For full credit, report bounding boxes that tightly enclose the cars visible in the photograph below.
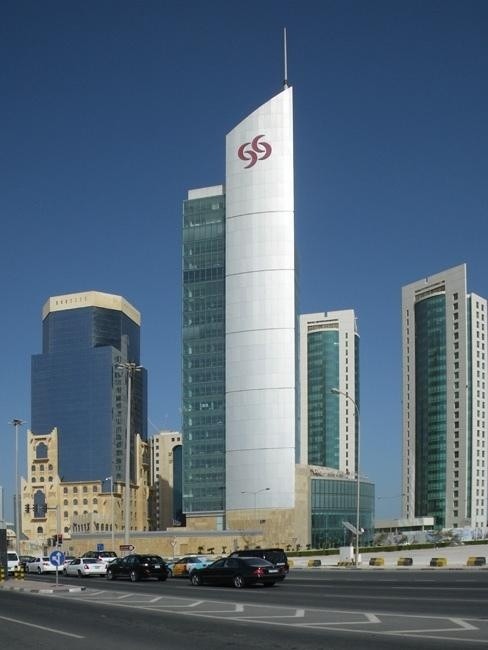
[188,557,285,588]
[7,551,231,582]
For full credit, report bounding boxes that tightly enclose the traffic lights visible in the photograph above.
[47,538,51,547]
[25,504,29,512]
[52,535,57,543]
[33,504,38,513]
[53,538,56,546]
[42,503,47,512]
[58,535,63,544]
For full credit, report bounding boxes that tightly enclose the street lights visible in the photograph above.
[105,475,115,552]
[330,389,361,569]
[240,487,271,548]
[377,493,406,545]
[8,418,27,556]
[116,362,144,557]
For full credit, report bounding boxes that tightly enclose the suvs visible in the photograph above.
[228,548,289,583]
